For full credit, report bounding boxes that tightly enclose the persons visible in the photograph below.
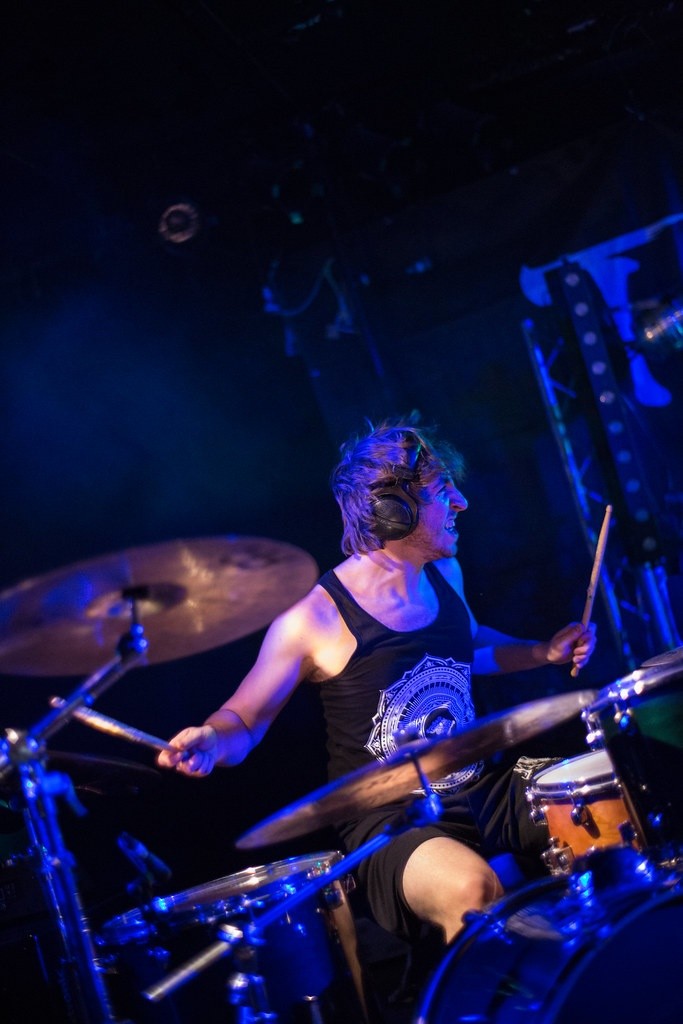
[152,419,599,945]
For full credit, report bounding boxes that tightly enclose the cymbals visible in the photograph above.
[1,539,319,677]
[234,692,594,850]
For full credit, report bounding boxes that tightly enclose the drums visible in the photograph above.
[412,849,683,1024]
[74,851,365,1024]
[525,748,647,876]
[587,646,683,868]
